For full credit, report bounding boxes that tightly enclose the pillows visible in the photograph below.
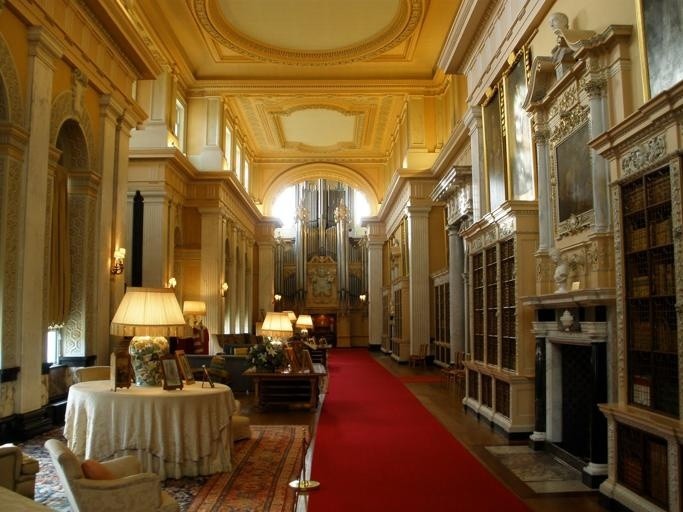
[81,460,116,480]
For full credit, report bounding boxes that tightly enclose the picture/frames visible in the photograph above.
[159,353,184,391]
[481,45,594,241]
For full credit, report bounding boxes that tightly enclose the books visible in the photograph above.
[623,175,677,416]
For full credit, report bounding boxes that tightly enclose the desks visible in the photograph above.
[62,380,237,481]
[241,363,327,412]
[309,344,333,367]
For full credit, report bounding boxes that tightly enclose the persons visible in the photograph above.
[549,247,568,293]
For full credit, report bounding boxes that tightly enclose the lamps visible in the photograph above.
[110,246,314,387]
[359,291,369,305]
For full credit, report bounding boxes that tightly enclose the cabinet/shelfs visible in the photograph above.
[460,199,539,441]
[380,276,410,362]
[587,80,683,512]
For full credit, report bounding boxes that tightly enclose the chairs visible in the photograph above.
[0,443,40,500]
[409,343,428,369]
[440,352,467,398]
[231,399,252,443]
[72,365,112,384]
[44,438,179,512]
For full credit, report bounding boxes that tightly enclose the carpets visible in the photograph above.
[17,424,311,512]
